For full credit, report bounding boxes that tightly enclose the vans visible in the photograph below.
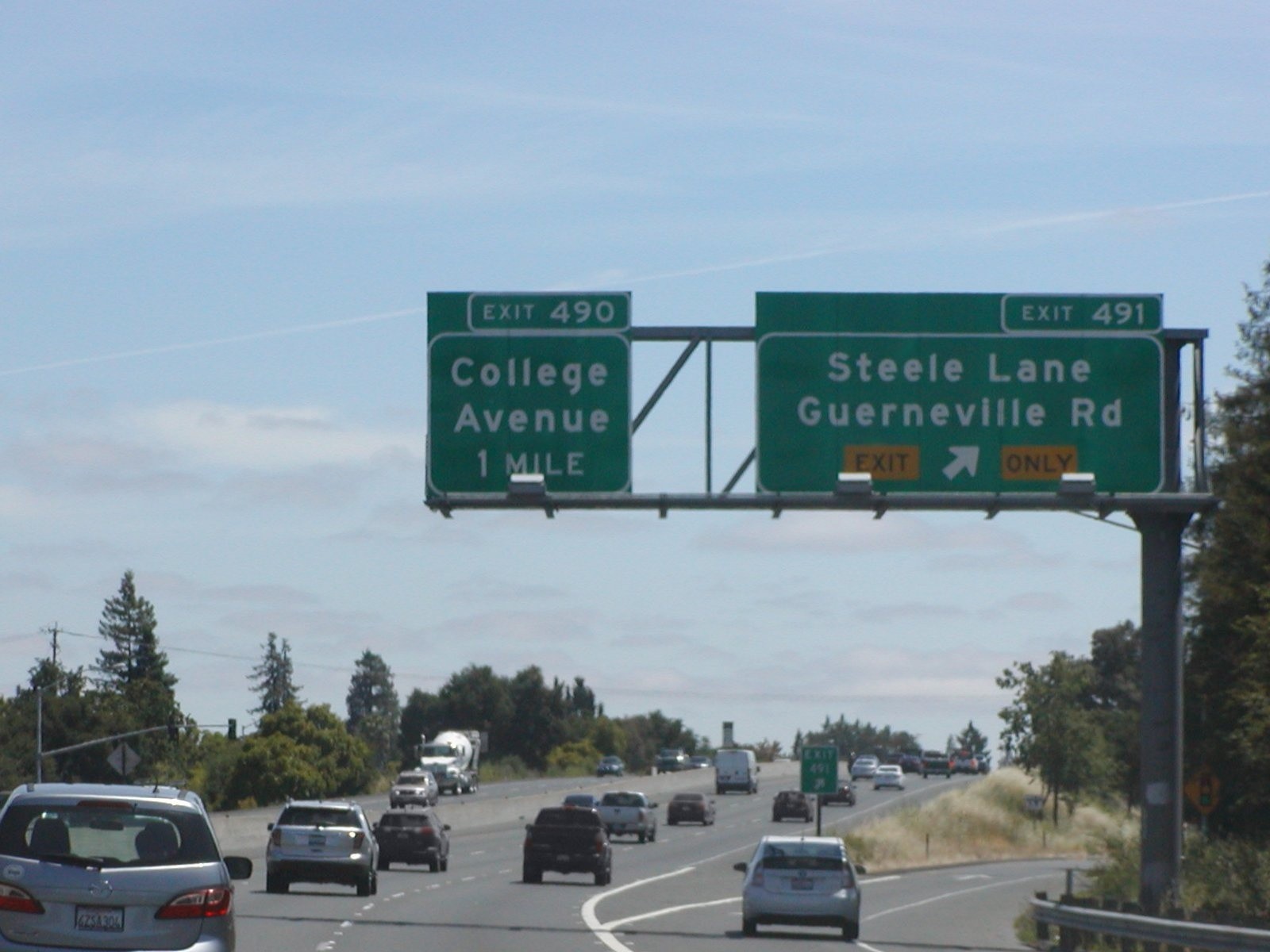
[715,750,761,795]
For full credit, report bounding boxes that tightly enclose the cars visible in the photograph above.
[822,780,857,806]
[667,794,719,826]
[772,790,816,823]
[596,755,627,777]
[562,796,598,815]
[851,749,989,789]
[735,835,867,937]
[689,755,712,769]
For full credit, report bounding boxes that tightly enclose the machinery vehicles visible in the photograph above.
[413,729,482,794]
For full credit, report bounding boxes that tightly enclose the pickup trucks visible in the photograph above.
[523,808,612,884]
[595,793,658,841]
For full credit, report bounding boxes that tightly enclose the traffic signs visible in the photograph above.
[753,331,1164,495]
[425,332,634,501]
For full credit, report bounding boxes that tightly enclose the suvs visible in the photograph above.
[389,766,439,809]
[266,798,380,894]
[372,808,451,873]
[655,750,690,774]
[0,781,252,951]
